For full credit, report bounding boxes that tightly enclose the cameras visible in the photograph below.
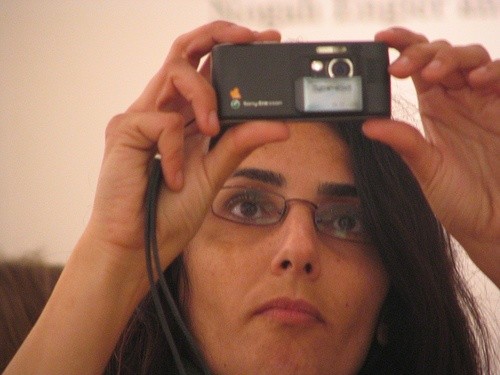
[210,39,393,122]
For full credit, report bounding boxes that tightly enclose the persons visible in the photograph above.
[0,21,500,374]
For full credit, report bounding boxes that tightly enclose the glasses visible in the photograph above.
[212,186,373,244]
